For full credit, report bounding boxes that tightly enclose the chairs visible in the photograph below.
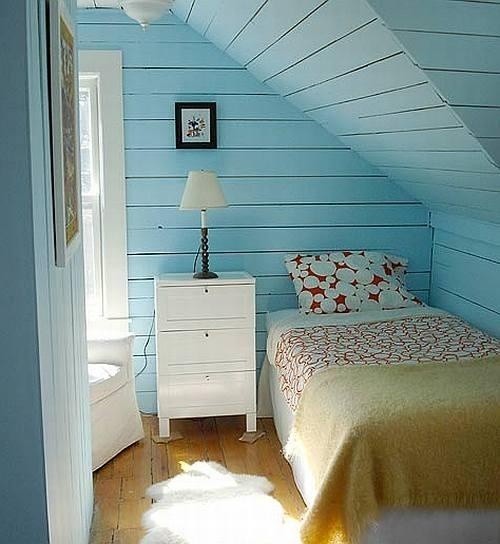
[86,332,145,471]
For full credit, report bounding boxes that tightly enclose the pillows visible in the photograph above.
[284,251,424,316]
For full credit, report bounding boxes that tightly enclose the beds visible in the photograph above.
[262,303,500,544]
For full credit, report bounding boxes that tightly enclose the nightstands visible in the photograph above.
[154,273,257,439]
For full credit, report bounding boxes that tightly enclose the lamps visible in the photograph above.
[117,0,176,27]
[179,170,227,279]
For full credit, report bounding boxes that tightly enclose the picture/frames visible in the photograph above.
[174,101,217,150]
[46,0,83,267]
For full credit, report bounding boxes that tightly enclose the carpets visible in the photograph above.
[141,460,303,544]
